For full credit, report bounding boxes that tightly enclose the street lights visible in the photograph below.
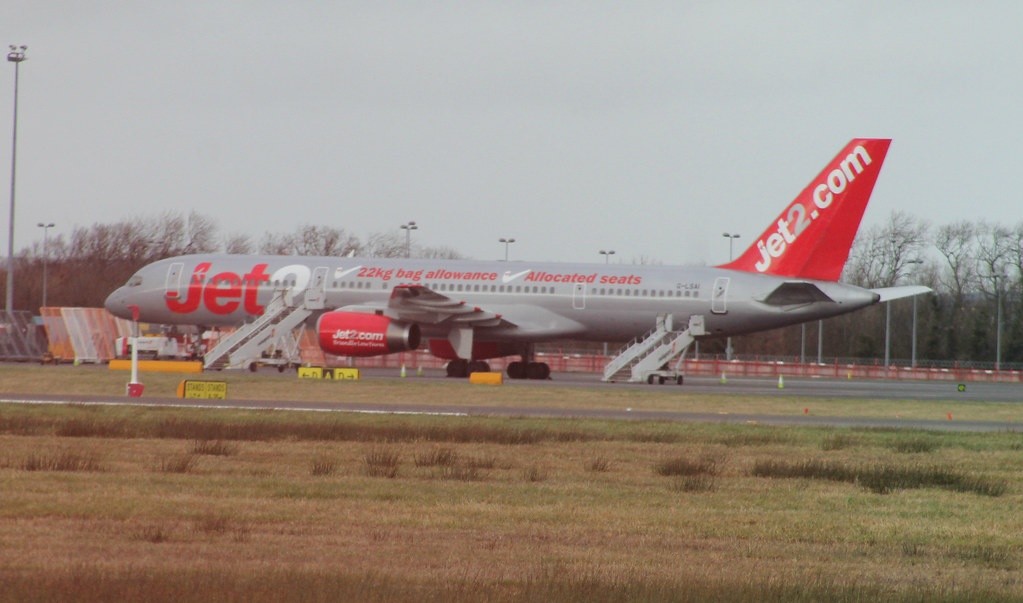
[36,221,55,307]
[907,258,928,367]
[723,232,741,260]
[500,238,515,261]
[993,273,1010,371]
[2,44,27,326]
[599,249,615,264]
[400,220,418,257]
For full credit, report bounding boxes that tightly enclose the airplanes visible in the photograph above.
[105,136,940,401]
